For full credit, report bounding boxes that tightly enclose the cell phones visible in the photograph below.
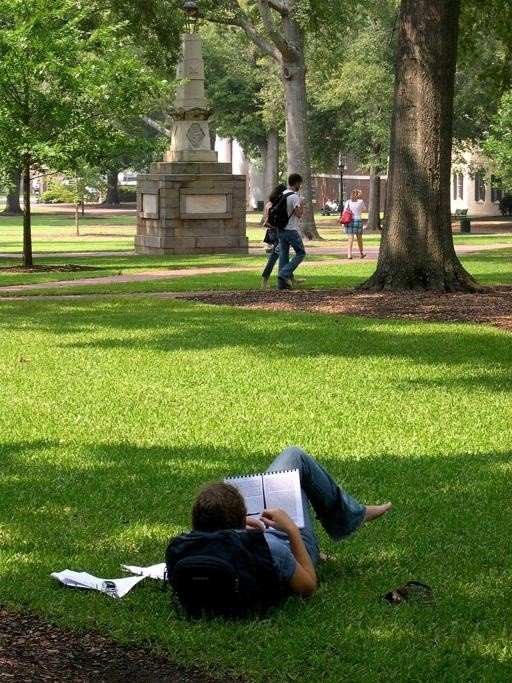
[101,581,117,593]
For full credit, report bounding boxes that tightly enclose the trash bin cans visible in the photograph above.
[461,217,470,233]
[255,200,265,211]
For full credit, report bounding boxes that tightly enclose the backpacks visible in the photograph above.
[268,192,295,228]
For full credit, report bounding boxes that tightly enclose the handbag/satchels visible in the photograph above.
[340,209,352,223]
[165,528,275,620]
[263,229,278,243]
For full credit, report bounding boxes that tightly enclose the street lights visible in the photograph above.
[160,0,219,161]
[338,161,346,218]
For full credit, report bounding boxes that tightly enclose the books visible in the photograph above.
[223,469,305,529]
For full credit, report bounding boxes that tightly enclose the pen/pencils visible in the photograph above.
[246,512,261,516]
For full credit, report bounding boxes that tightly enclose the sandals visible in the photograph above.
[380,579,436,606]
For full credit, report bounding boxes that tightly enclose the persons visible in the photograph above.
[33,173,40,196]
[261,184,306,289]
[277,174,305,290]
[321,198,332,216]
[342,188,367,259]
[321,200,337,215]
[192,448,393,599]
